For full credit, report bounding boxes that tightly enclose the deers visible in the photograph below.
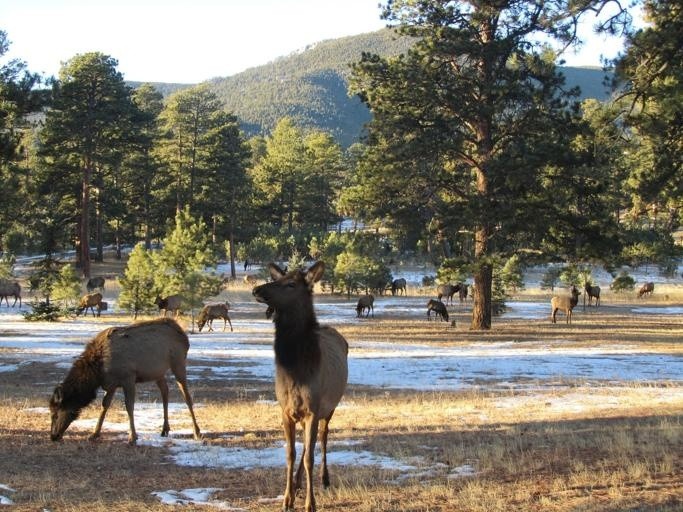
[195,301,233,333]
[550,280,602,325]
[49,317,203,446]
[636,283,655,300]
[73,274,105,317]
[426,282,469,323]
[252,260,350,512]
[0,282,22,309]
[355,294,375,317]
[154,293,184,317]
[391,278,407,297]
